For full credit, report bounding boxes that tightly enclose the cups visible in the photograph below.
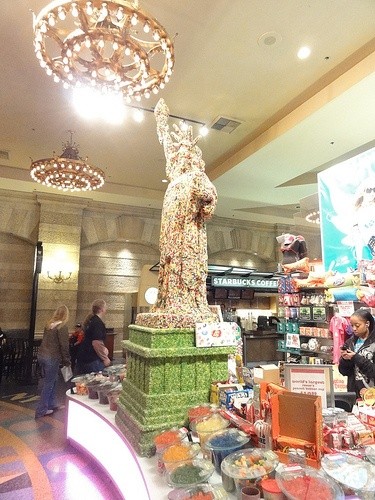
[240,486,260,500]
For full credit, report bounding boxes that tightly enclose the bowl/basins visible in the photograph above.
[71,364,127,411]
[154,402,375,500]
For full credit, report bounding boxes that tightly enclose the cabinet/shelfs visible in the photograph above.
[273,271,369,365]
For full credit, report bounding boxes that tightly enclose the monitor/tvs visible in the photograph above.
[257,316,267,328]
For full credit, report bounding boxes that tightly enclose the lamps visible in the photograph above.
[47,270,73,284]
[31,1,175,101]
[30,130,104,191]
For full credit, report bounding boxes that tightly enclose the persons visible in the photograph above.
[34,304,73,419]
[66,323,85,359]
[338,309,375,398]
[77,299,111,376]
[0,328,7,388]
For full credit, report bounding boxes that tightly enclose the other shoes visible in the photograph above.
[35,410,53,418]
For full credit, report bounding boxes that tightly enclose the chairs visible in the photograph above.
[0,338,45,385]
